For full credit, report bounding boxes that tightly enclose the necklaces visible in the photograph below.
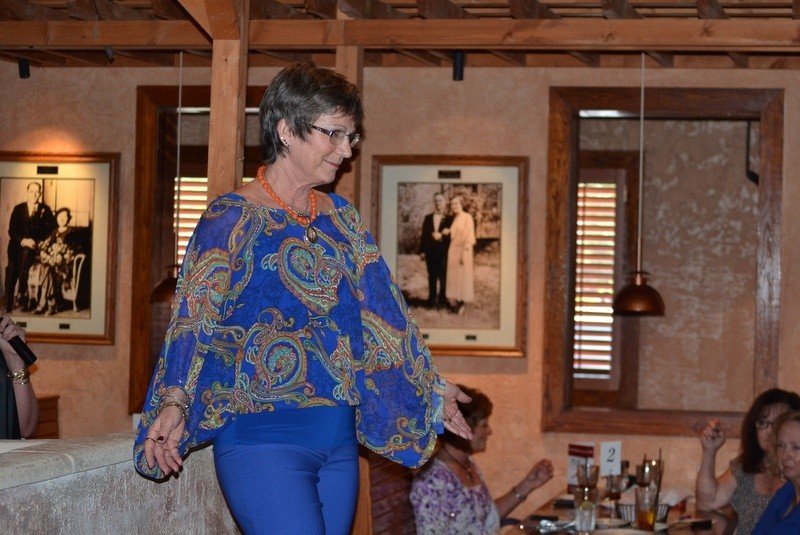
[256,165,317,244]
[441,445,473,479]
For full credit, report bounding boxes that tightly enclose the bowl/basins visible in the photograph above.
[618,503,668,528]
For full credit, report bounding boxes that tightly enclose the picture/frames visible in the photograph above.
[0,150,121,345]
[371,153,530,358]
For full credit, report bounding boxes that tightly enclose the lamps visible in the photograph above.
[148,51,183,306]
[613,54,664,316]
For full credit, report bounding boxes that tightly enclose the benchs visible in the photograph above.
[351,441,441,535]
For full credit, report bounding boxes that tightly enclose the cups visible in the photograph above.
[572,487,600,535]
[635,458,664,531]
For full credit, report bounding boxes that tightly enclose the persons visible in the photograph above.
[419,192,476,314]
[133,59,473,535]
[0,317,40,440]
[695,387,800,535]
[409,383,555,535]
[3,182,81,317]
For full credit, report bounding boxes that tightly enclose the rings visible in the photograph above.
[146,437,156,443]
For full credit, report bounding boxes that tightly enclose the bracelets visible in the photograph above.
[156,394,189,423]
[7,367,32,384]
[512,487,526,501]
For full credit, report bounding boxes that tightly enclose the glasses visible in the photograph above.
[309,125,360,147]
[755,419,775,430]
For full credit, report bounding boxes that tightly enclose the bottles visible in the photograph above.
[616,460,633,519]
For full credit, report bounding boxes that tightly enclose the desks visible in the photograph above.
[504,490,738,535]
